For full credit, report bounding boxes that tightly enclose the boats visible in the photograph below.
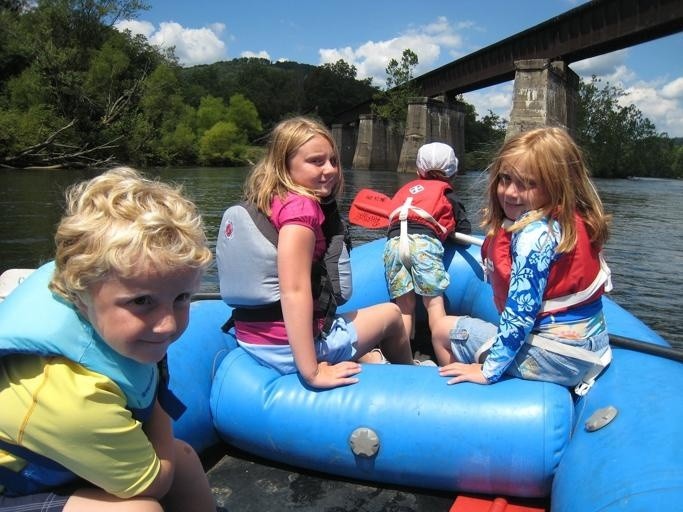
[164,227,683,512]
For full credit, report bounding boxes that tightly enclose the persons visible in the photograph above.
[1,162,225,512]
[382,141,473,363]
[213,111,437,393]
[428,124,616,400]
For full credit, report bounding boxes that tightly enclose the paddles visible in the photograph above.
[349,189,485,245]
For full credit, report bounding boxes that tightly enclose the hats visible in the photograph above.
[416,142,459,178]
